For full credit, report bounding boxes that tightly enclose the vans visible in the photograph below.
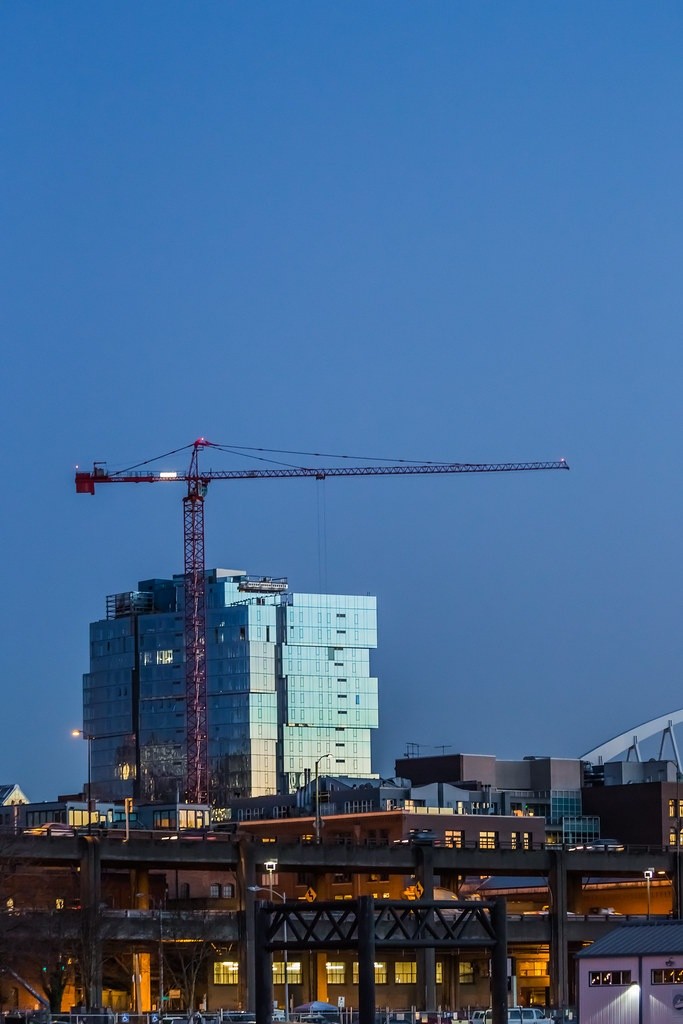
[470,1008,555,1024]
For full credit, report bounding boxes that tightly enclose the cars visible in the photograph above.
[65,898,82,910]
[584,839,624,851]
[22,823,74,837]
[409,831,441,847]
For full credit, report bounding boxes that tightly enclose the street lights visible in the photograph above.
[316,754,333,844]
[72,729,92,834]
[265,859,279,983]
[137,893,163,1014]
[248,885,288,1021]
[658,870,680,920]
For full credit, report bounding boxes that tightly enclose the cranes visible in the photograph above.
[76,437,569,808]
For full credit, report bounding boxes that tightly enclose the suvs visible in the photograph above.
[589,906,624,916]
[109,820,146,830]
